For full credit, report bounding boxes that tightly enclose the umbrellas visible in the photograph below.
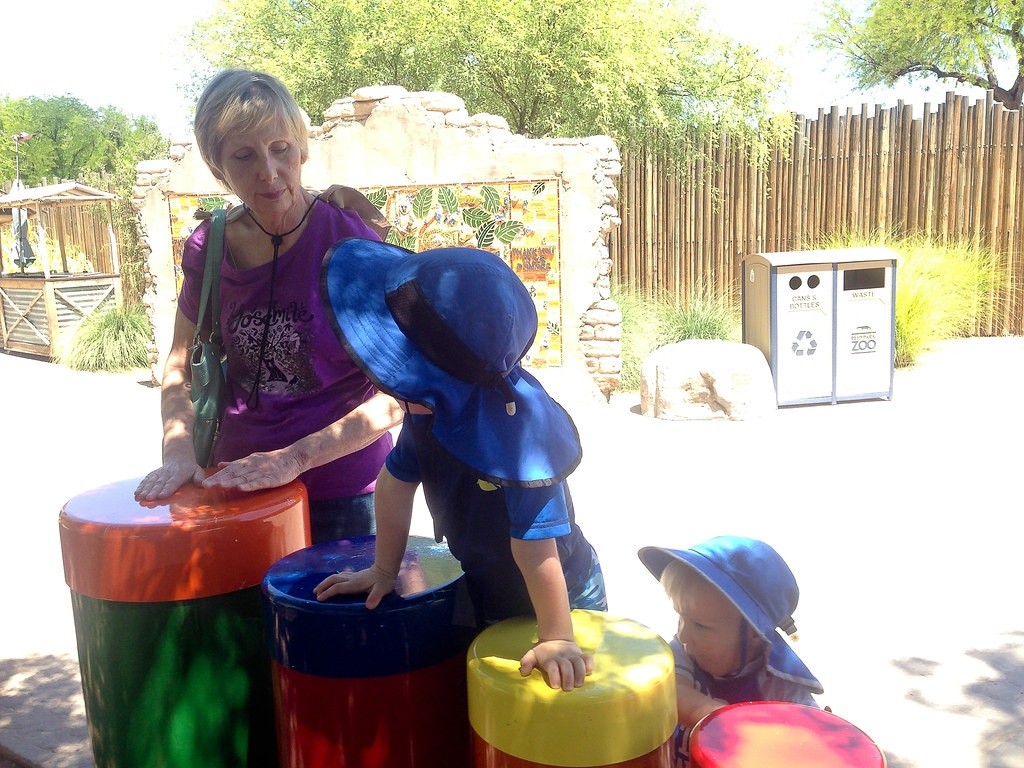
[9,178,36,273]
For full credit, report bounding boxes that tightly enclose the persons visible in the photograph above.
[135,70,405,544]
[637,535,823,768]
[312,236,608,691]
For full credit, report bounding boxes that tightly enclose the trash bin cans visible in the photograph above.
[737,245,901,410]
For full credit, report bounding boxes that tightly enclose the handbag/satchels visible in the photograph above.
[190,211,226,469]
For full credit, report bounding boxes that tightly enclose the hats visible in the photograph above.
[638,533,824,696]
[319,233,581,489]
